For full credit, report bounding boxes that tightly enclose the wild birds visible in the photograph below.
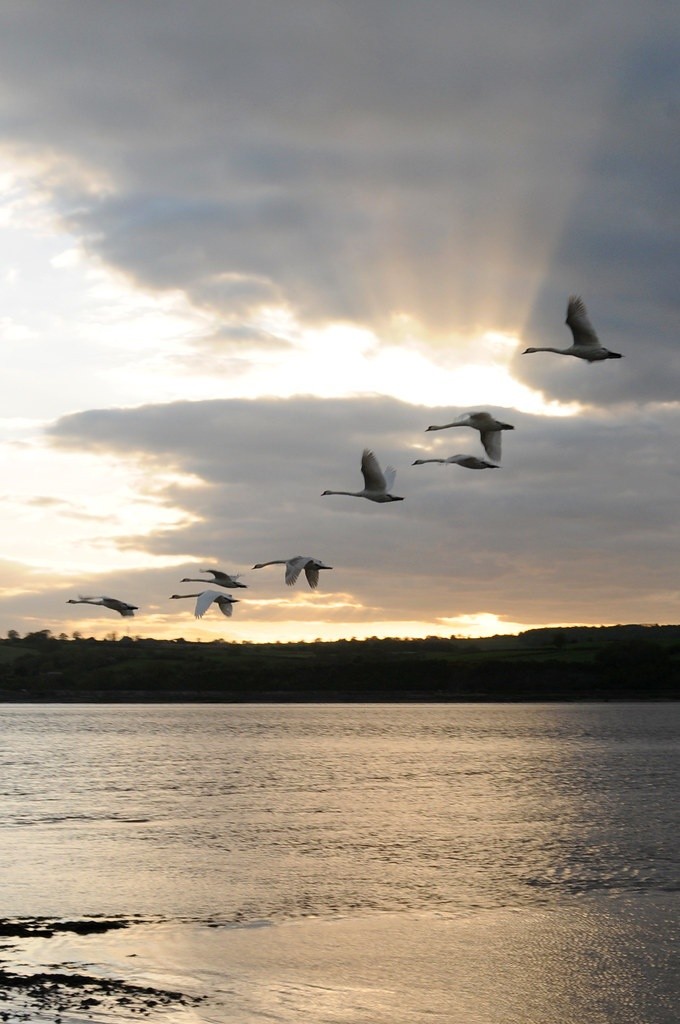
[169,590,242,619]
[320,447,405,503]
[521,289,625,365]
[180,568,250,589]
[67,596,137,618]
[251,555,333,589]
[424,410,516,462]
[411,453,500,470]
